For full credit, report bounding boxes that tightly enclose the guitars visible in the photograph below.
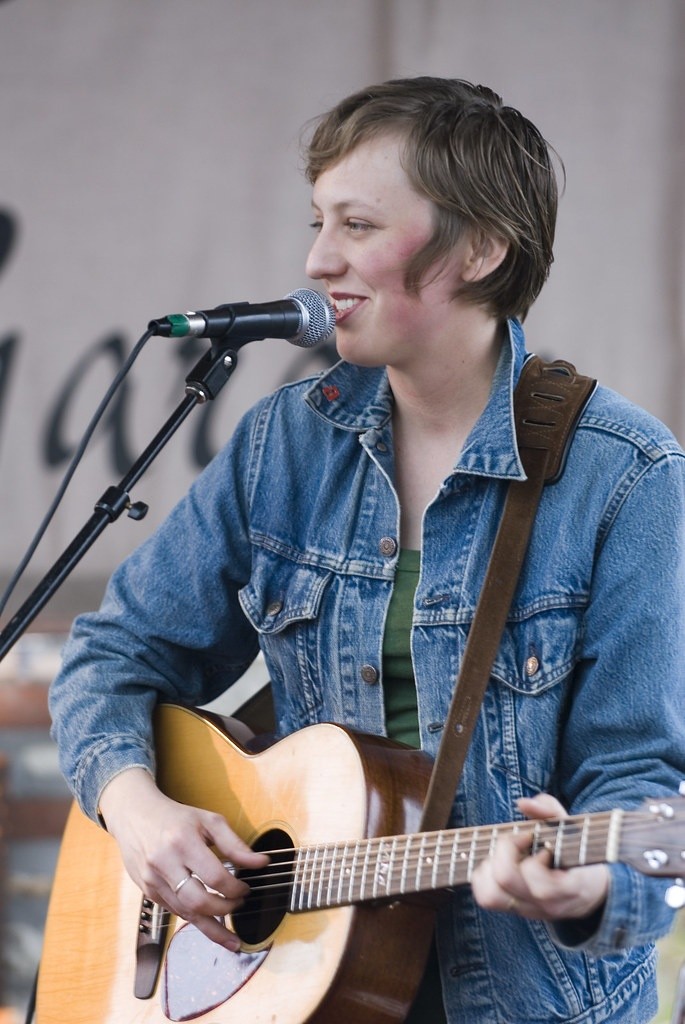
[24,700,685,1024]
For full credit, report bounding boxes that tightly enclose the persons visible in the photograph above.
[43,72,685,1022]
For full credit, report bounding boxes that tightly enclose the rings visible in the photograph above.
[174,874,192,896]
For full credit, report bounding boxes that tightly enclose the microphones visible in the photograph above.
[150,288,336,347]
